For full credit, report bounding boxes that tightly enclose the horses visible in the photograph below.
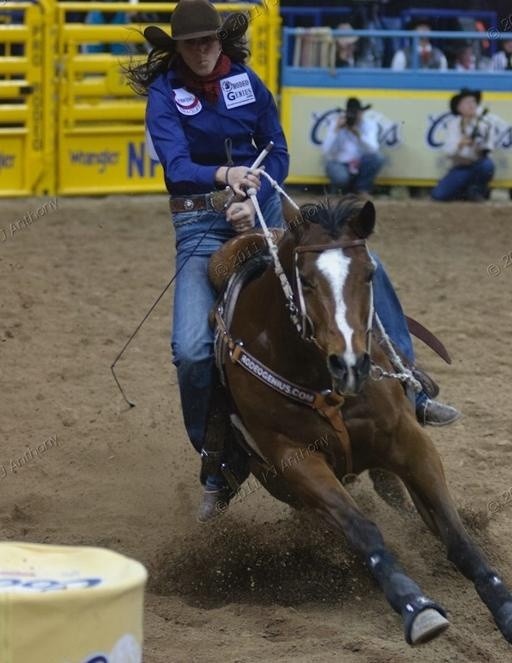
[207,188,512,651]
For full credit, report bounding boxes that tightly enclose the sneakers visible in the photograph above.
[197,457,250,524]
[417,396,461,427]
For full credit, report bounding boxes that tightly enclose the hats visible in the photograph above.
[450,87,481,116]
[144,1,249,52]
[337,98,371,112]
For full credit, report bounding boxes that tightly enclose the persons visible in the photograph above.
[453,47,488,70]
[353,0,411,69]
[432,86,499,203]
[145,1,462,534]
[333,21,369,67]
[320,96,390,198]
[389,15,446,69]
[490,39,511,69]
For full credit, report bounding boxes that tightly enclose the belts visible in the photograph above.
[169,191,230,214]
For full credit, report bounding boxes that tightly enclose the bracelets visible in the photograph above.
[223,165,235,183]
